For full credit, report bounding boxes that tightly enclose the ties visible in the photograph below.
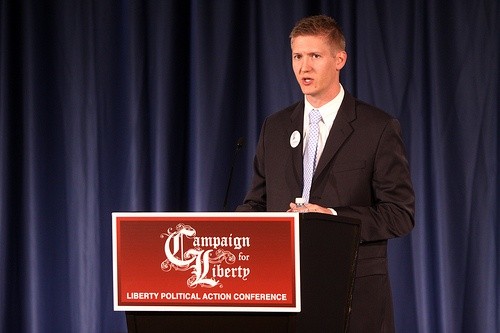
[302,109,322,204]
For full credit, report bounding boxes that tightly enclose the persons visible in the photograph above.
[235,16,415,333]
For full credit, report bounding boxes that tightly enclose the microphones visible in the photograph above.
[223,139,243,210]
